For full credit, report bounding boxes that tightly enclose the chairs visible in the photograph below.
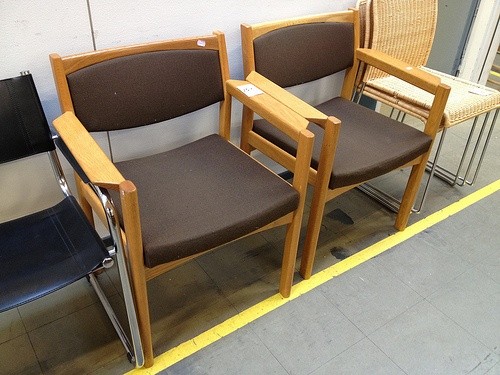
[44,24,321,373]
[1,64,148,375]
[348,0,500,219]
[235,5,450,284]
